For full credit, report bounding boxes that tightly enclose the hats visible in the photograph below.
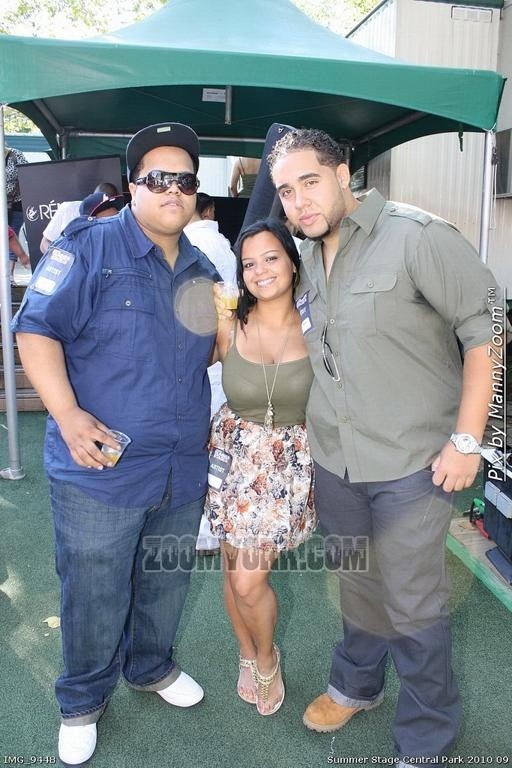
[126,123,200,183]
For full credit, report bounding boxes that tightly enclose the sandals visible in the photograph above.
[237,642,285,717]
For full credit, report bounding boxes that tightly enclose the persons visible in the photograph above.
[211,217,320,719]
[40,182,118,255]
[229,156,262,199]
[197,192,216,220]
[182,193,241,299]
[267,126,512,768]
[9,118,226,768]
[8,225,29,274]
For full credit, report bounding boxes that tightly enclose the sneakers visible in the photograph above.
[302,693,382,733]
[58,723,97,765]
[155,670,204,708]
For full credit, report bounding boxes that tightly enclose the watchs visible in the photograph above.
[448,432,484,456]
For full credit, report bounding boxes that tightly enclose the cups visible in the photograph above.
[214,280,240,310]
[101,429,132,469]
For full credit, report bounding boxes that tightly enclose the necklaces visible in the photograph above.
[256,304,296,436]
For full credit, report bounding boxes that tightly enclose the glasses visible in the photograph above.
[319,321,341,384]
[132,170,200,195]
